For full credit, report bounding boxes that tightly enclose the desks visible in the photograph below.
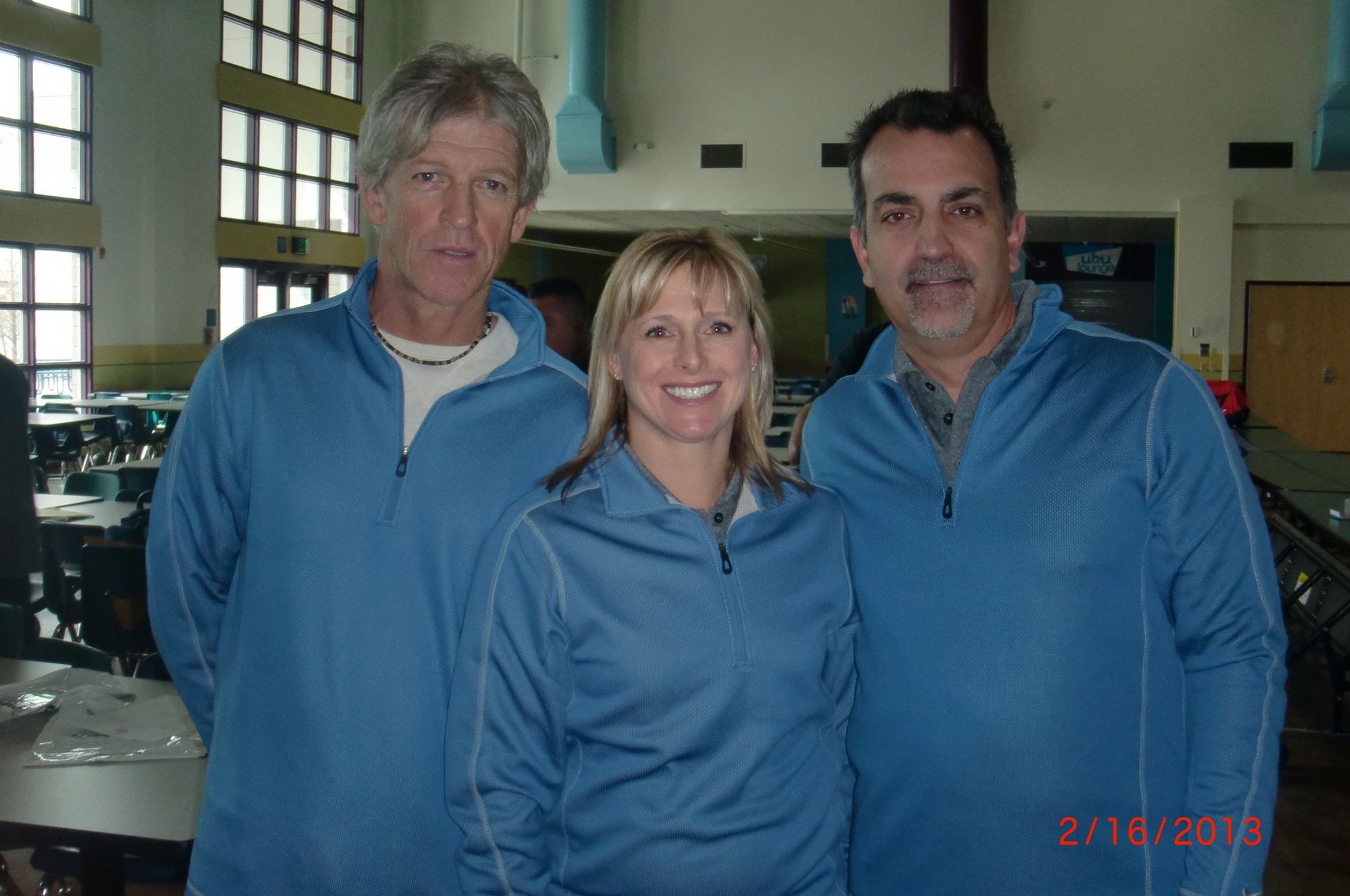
[0,398,208,896]
[88,389,184,398]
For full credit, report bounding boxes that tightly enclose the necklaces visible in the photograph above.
[370,316,491,366]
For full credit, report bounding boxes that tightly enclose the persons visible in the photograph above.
[801,88,1288,896]
[786,347,866,467]
[519,277,589,372]
[443,225,861,896]
[144,38,591,896]
[0,355,40,599]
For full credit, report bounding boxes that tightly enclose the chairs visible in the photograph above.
[27,392,185,681]
